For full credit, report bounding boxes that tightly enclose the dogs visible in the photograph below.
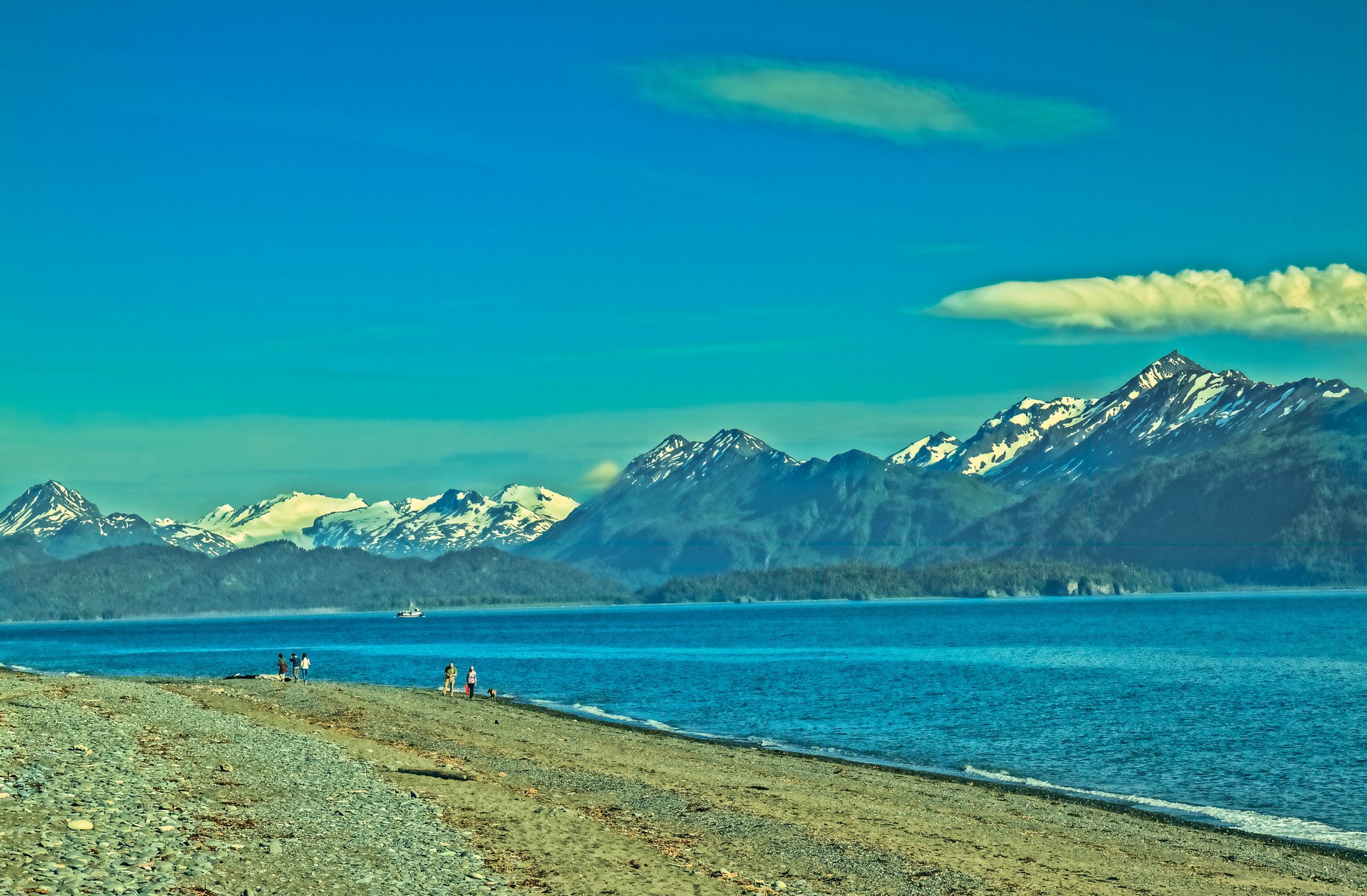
[487,687,497,702]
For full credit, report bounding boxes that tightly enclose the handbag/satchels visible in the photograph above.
[286,666,287,667]
[464,685,469,694]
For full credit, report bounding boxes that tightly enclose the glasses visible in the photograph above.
[470,670,473,670]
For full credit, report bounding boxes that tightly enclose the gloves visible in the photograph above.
[475,684,477,686]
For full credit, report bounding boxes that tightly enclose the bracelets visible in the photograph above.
[475,683,476,684]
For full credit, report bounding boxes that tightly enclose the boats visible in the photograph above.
[396,596,424,617]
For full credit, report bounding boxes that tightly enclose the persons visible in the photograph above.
[289,653,301,683]
[277,653,289,682]
[442,663,457,697]
[300,653,310,685]
[466,666,477,700]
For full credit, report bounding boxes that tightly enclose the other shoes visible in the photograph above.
[450,694,453,697]
[297,678,299,683]
[295,681,297,683]
[304,680,307,685]
[443,694,445,696]
[471,698,473,700]
[281,678,284,682]
